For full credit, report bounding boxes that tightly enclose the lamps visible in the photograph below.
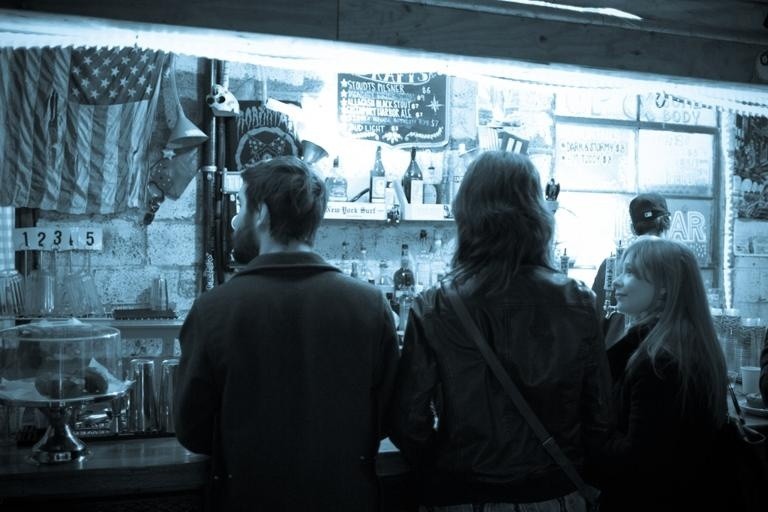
[166,53,209,151]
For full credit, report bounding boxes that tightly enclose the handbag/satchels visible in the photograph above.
[728,380,766,474]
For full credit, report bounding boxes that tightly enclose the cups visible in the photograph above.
[22,270,57,318]
[158,358,181,440]
[739,365,761,396]
[149,274,169,313]
[63,271,104,318]
[727,369,738,390]
[127,359,162,439]
[0,267,26,319]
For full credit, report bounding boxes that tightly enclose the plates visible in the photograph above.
[737,398,768,420]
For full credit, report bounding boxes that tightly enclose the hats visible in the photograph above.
[630,193,670,222]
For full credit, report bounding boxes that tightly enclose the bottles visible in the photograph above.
[338,228,459,355]
[369,147,387,203]
[423,164,442,204]
[450,153,481,206]
[325,153,350,203]
[458,143,474,168]
[400,146,422,204]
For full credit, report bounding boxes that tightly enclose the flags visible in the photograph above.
[0,45,180,215]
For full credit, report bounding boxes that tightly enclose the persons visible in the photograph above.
[395,150,605,512]
[172,157,401,500]
[610,238,727,511]
[591,192,672,327]
